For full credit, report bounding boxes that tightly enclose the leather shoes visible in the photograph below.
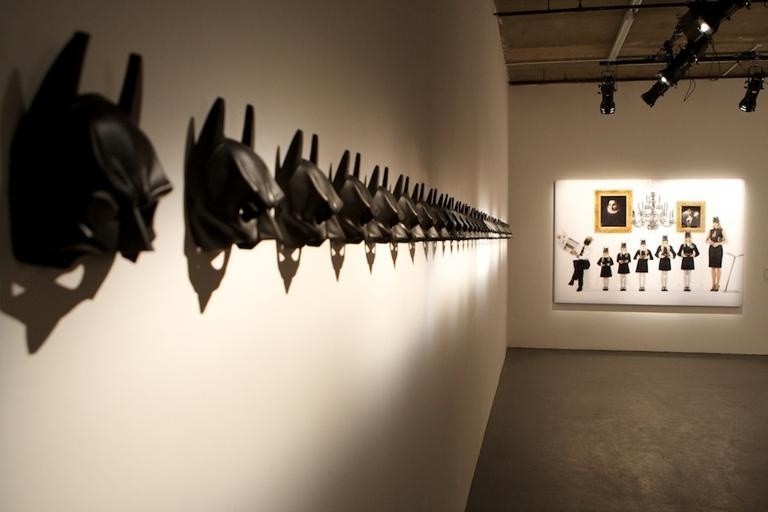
[577,287,582,291]
[603,286,691,291]
[569,279,574,285]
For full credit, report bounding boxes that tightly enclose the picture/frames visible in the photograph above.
[676,201,706,233]
[595,190,633,233]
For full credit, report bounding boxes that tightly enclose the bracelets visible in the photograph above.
[576,253,579,257]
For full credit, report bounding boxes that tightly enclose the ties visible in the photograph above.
[580,247,585,256]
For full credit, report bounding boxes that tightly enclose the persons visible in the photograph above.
[634,239,654,291]
[678,231,700,291]
[654,235,677,291]
[704,216,728,291]
[597,246,614,291]
[616,242,631,291]
[567,234,593,293]
[682,207,700,227]
[602,197,625,227]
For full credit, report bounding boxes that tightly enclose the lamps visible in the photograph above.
[632,192,675,231]
[597,0,768,115]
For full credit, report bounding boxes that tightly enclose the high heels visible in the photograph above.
[710,284,720,291]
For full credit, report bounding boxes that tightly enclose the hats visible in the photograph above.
[713,216,719,223]
[603,231,691,253]
